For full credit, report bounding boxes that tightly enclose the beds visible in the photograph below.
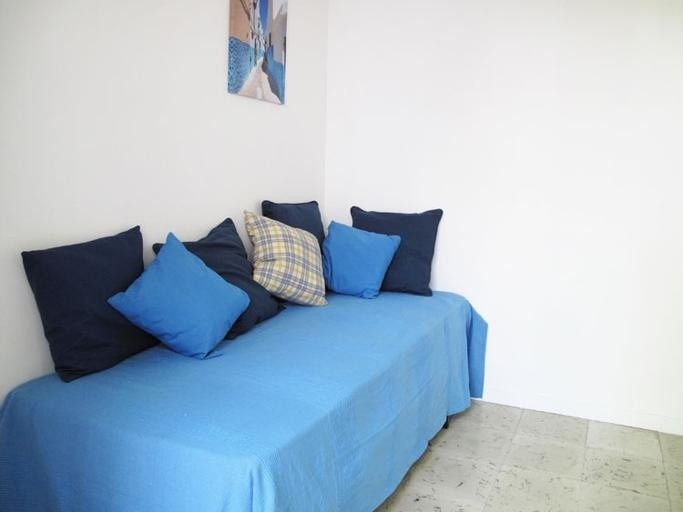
[0,289,486,512]
